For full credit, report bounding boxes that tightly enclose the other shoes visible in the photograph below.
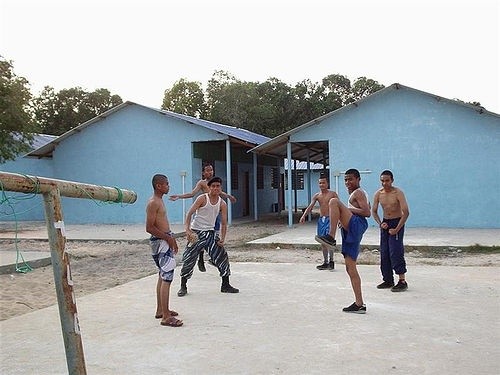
[316,263,329,269]
[327,261,334,270]
[198,260,206,272]
[391,281,408,291]
[343,302,366,313]
[377,281,395,289]
[315,234,336,249]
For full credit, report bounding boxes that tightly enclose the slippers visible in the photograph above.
[160,316,183,327]
[155,310,178,318]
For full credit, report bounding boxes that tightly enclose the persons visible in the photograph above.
[372,170,409,292]
[315,169,372,314]
[169,163,236,273]
[146,174,183,327]
[299,176,339,270]
[178,177,239,297]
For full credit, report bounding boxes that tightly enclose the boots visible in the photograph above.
[221,276,239,293]
[178,279,187,296]
